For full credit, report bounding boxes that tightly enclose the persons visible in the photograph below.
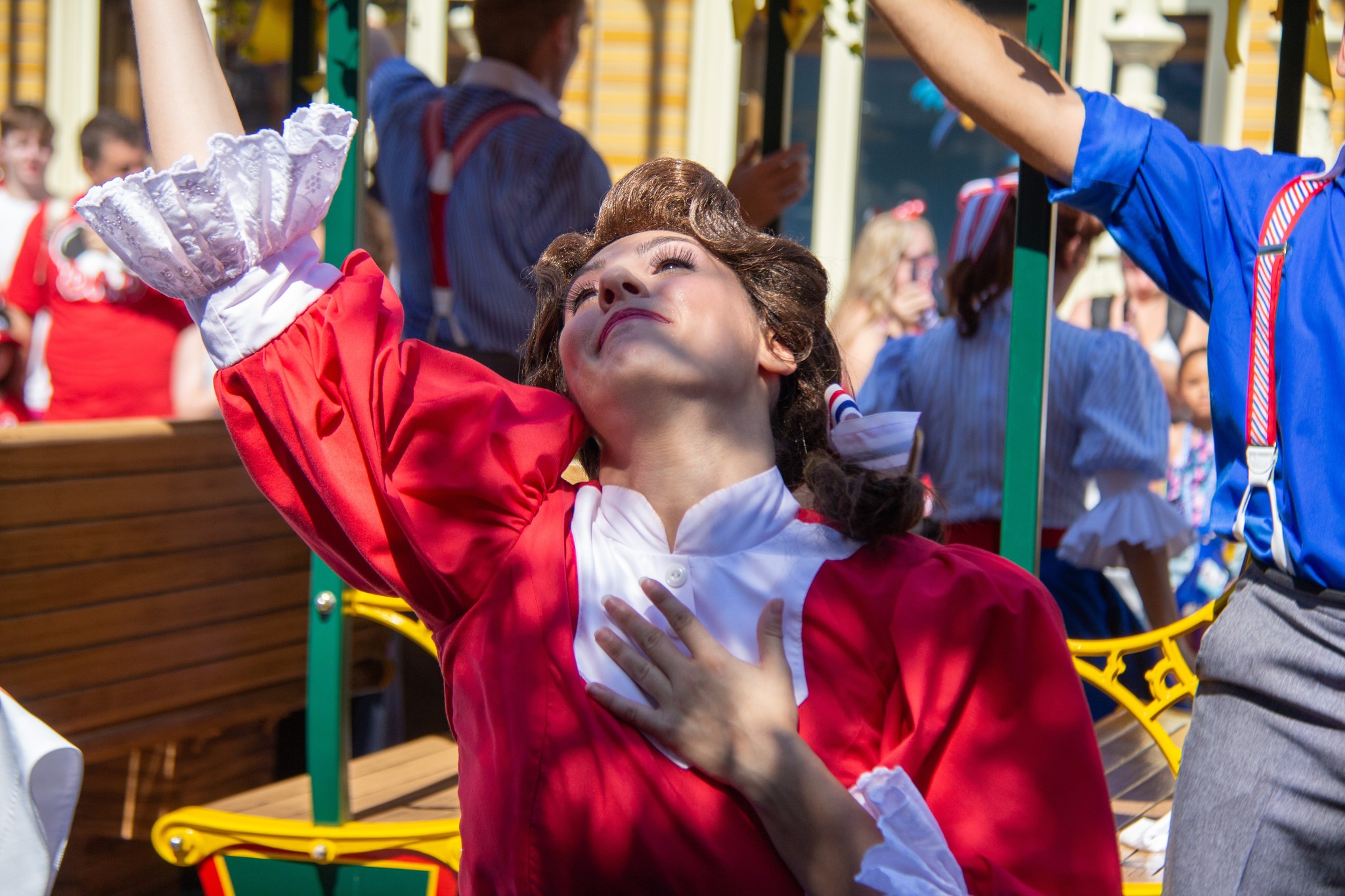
[832,207,943,397]
[365,2,615,748]
[0,107,71,314]
[0,304,35,424]
[73,0,1124,895]
[48,117,217,422]
[1064,254,1215,610]
[866,0,1345,896]
[856,154,1197,722]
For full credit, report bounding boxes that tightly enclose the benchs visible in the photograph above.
[1066,545,1252,896]
[149,590,462,896]
[0,421,403,896]
